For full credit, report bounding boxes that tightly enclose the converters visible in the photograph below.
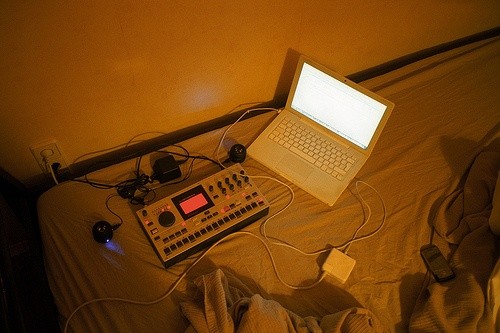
[154,156,182,183]
[323,248,356,283]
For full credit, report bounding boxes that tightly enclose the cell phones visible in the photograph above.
[421,246,455,282]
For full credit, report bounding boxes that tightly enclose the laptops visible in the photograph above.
[244,56,396,206]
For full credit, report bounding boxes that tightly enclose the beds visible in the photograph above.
[37,26,500,333]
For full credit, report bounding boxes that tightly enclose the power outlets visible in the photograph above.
[30,140,71,184]
